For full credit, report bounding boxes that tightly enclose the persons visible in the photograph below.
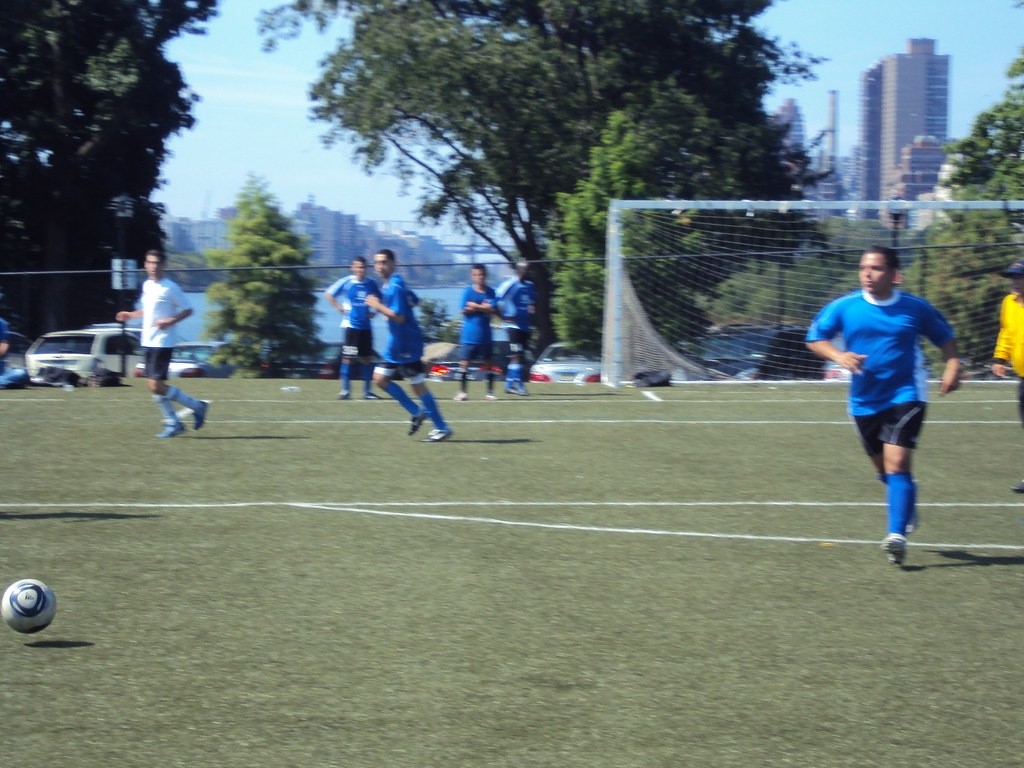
[323,256,385,400]
[115,250,209,437]
[365,249,454,442]
[992,262,1024,494]
[455,264,501,401]
[804,244,962,567]
[496,259,538,396]
[0,319,9,359]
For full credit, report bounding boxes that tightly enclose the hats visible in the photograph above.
[997,259,1024,277]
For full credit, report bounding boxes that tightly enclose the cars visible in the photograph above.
[0,331,31,386]
[283,344,383,380]
[531,342,602,384]
[138,341,238,378]
[425,346,504,384]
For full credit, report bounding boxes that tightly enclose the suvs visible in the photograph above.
[24,327,145,386]
[690,325,823,387]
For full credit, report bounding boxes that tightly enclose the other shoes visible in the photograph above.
[504,386,530,396]
[454,391,469,401]
[881,532,907,565]
[1010,481,1024,493]
[485,394,499,400]
[155,421,185,438]
[904,505,919,533]
[407,406,432,435]
[363,391,381,400]
[338,389,352,399]
[420,423,452,443]
[192,400,208,430]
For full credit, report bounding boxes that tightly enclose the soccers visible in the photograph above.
[0,578,57,634]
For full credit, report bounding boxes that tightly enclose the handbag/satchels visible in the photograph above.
[37,366,80,386]
